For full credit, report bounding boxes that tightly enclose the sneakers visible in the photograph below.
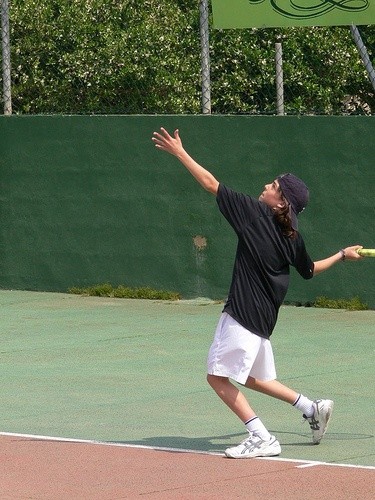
[224,428,281,459]
[303,399,334,445]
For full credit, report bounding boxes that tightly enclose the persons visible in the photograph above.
[151,126,363,459]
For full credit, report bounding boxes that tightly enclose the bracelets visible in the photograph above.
[339,248,346,262]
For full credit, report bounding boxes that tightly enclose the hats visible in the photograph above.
[277,171,309,232]
